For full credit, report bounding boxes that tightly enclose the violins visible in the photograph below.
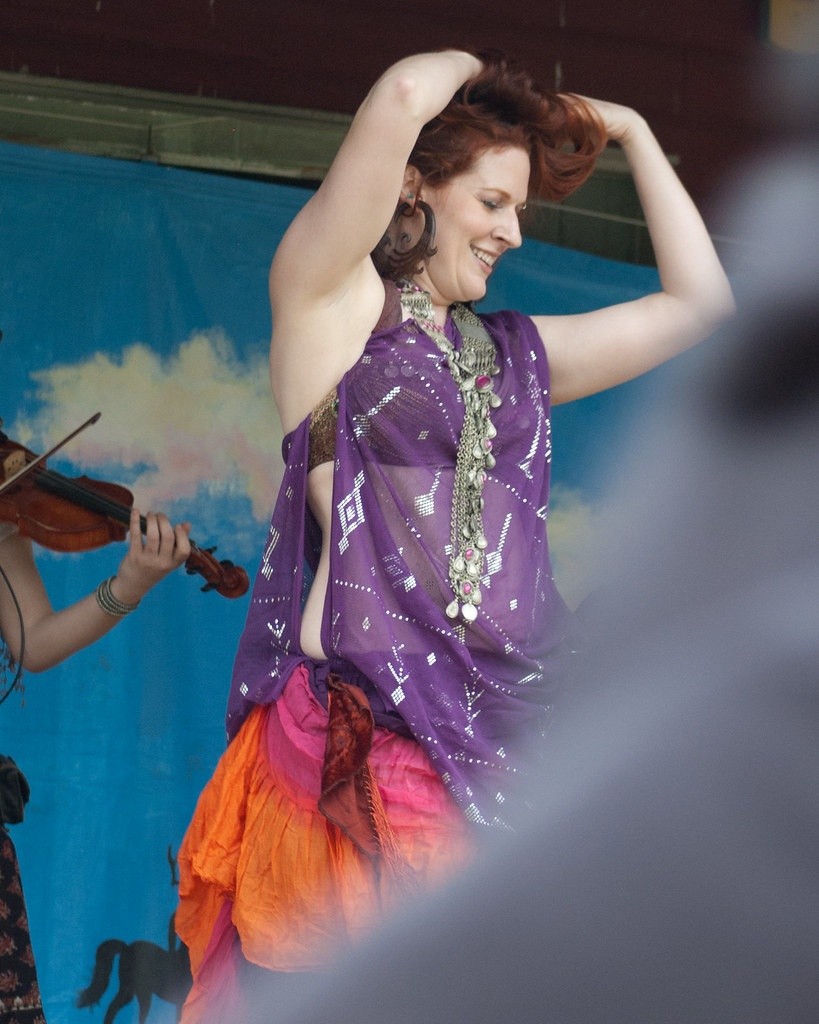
[0,437,250,599]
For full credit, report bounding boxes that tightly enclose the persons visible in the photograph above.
[173,49,737,1024]
[0,511,191,1024]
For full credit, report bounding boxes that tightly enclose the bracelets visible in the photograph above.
[96,576,140,617]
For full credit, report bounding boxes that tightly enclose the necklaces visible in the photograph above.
[387,281,502,622]
